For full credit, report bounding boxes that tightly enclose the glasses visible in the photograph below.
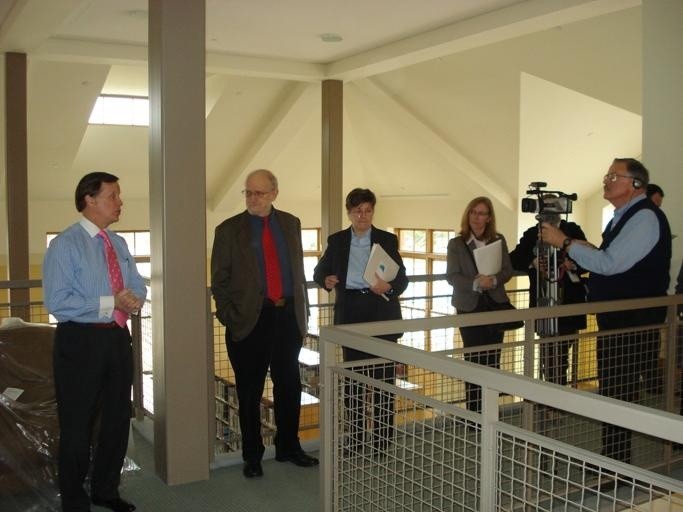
[604,172,636,183]
[241,189,273,198]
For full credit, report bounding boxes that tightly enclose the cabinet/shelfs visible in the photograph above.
[216,369,320,440]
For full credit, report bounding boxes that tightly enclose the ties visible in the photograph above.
[261,215,285,303]
[97,230,129,329]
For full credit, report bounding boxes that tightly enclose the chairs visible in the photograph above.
[390,373,426,423]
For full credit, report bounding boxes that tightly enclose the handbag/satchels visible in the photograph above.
[487,300,524,331]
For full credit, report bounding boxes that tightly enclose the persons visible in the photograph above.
[446,197,524,432]
[536,159,673,477]
[675,264,683,326]
[640,184,664,394]
[211,169,320,478]
[314,188,409,455]
[509,194,590,415]
[42,171,147,512]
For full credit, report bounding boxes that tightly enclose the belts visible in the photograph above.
[93,321,127,330]
[263,296,293,307]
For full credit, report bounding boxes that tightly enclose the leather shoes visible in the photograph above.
[275,449,320,467]
[345,288,372,295]
[242,457,264,477]
[90,495,137,512]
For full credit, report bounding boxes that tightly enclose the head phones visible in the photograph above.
[633,177,644,190]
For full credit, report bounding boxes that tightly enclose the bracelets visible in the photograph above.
[386,289,393,295]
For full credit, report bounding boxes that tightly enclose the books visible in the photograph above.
[214,376,276,455]
[363,242,400,302]
[299,363,318,396]
[473,238,502,276]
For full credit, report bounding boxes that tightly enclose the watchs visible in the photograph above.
[563,237,573,250]
